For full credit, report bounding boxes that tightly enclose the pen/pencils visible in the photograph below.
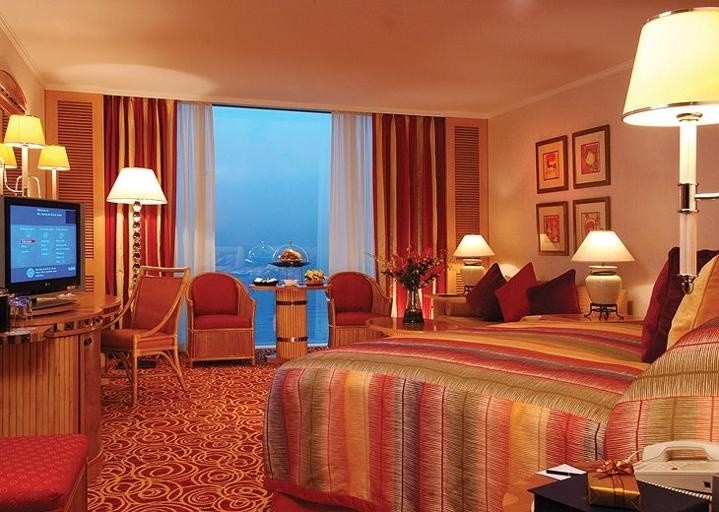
[547,470,578,476]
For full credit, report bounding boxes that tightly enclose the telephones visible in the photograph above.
[633,440,718,494]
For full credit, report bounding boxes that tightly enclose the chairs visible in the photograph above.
[101,262,257,408]
[324,271,394,349]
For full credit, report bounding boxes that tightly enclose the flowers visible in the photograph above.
[372,241,453,293]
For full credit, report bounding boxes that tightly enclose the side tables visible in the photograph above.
[249,282,331,364]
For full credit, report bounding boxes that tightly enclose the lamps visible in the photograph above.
[105,167,170,291]
[621,6,719,293]
[571,229,635,321]
[0,112,71,201]
[451,232,496,296]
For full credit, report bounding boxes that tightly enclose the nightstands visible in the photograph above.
[501,461,712,512]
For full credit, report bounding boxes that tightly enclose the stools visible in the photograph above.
[1,433,91,511]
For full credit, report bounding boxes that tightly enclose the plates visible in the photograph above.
[253,281,278,286]
[269,261,311,266]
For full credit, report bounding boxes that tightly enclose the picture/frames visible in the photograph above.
[534,124,612,258]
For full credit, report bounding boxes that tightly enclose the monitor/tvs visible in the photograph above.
[0,195,85,294]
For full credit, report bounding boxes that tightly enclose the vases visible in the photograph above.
[402,290,425,327]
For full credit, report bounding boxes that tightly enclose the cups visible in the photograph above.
[0,295,10,332]
[10,296,33,333]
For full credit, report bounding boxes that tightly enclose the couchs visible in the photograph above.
[431,279,642,329]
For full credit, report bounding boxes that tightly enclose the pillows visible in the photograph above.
[639,246,719,363]
[464,261,581,323]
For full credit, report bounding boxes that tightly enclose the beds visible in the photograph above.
[264,319,718,512]
